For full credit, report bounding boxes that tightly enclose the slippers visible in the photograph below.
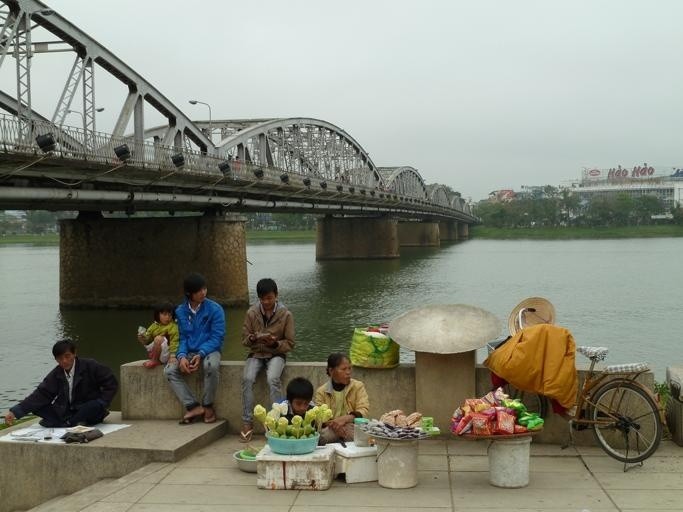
[239,423,255,443]
[202,395,216,423]
[178,412,204,424]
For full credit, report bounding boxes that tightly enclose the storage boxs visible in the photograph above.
[325,441,379,485]
[255,444,335,491]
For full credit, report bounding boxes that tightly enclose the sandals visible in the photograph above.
[144,361,161,368]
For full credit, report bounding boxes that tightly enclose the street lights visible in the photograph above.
[188,101,212,142]
[67,107,106,147]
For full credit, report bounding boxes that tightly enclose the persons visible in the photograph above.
[239,276,295,444]
[137,298,179,370]
[314,351,370,447]
[4,336,119,427]
[162,272,226,426]
[270,374,323,435]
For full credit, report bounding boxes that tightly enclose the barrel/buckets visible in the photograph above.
[486,438,532,488]
[376,438,418,489]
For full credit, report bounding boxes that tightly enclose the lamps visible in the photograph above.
[387,194,478,221]
[370,191,375,197]
[360,189,366,195]
[113,143,132,161]
[320,181,328,189]
[348,187,354,193]
[379,193,384,199]
[303,178,312,187]
[336,185,343,191]
[171,153,186,168]
[280,173,289,183]
[36,132,57,154]
[218,161,231,173]
[253,167,264,179]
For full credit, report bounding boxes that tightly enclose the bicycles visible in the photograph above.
[492,306,662,474]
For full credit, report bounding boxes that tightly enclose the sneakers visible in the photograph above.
[39,418,60,427]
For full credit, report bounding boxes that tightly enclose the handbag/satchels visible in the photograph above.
[350,327,400,369]
